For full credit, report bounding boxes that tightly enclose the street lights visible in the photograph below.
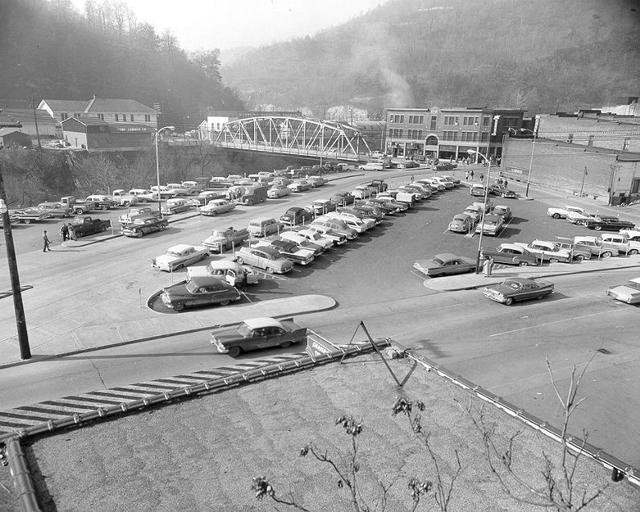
[467,149,491,274]
[155,126,176,216]
[508,128,517,138]
[521,128,535,197]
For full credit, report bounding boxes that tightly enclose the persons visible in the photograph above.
[61,223,77,242]
[242,268,251,291]
[42,230,52,252]
[411,174,415,183]
[449,153,509,186]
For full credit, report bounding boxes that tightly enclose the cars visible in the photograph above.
[412,252,476,276]
[204,228,249,252]
[484,276,555,306]
[448,183,640,267]
[211,316,309,358]
[160,277,242,312]
[235,150,465,274]
[184,259,260,287]
[151,244,210,272]
[0,172,240,237]
[606,276,640,305]
